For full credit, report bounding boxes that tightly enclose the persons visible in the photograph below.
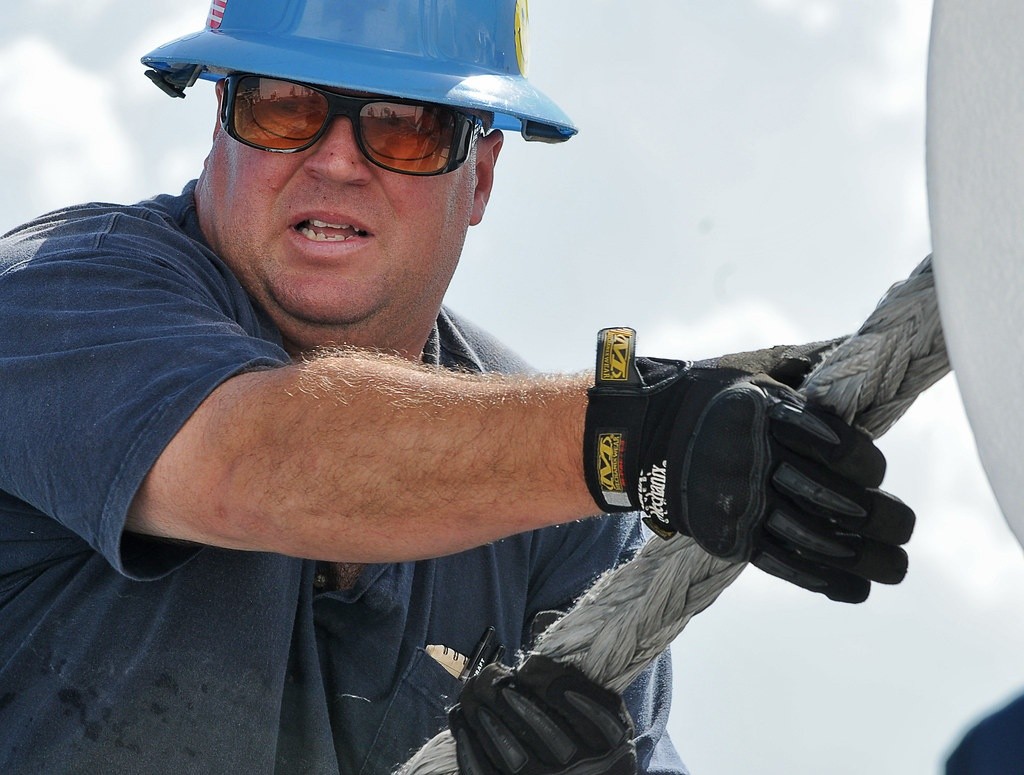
[1,0,914,775]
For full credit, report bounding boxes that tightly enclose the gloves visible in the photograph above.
[445,652,643,775]
[582,326,917,605]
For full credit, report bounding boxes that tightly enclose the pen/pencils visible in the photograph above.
[459,625,496,683]
[492,644,506,664]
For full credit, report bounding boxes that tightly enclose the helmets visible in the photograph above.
[143,0,579,147]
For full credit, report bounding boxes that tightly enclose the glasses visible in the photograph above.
[196,70,487,176]
